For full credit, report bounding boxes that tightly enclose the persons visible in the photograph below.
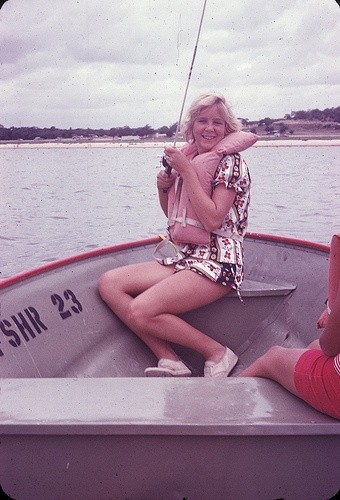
[96,89,252,378]
[235,231,340,420]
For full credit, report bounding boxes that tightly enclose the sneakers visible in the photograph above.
[203,345,238,377]
[144,358,192,377]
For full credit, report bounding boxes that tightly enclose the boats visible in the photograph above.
[0,232,340,500]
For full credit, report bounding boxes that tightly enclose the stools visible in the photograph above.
[225,278,292,299]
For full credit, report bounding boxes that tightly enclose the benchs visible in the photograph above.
[0,377,340,500]
